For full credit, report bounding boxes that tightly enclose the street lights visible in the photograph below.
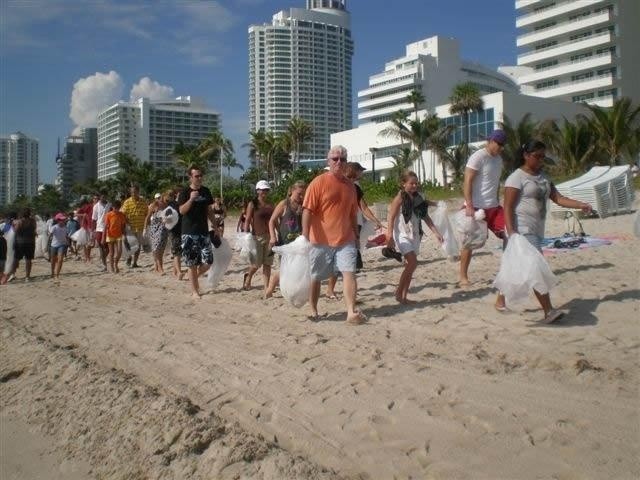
[369,148,377,183]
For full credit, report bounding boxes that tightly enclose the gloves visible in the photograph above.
[143,229,147,239]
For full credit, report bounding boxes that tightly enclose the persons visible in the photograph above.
[455,129,507,289]
[494,140,595,325]
[236,143,446,325]
[0,164,225,301]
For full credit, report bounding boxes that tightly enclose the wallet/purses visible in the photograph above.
[209,232,221,248]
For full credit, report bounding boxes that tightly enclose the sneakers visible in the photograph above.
[496,303,507,311]
[544,311,564,324]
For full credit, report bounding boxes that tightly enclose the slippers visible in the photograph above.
[382,247,402,262]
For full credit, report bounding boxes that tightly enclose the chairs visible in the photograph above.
[550,209,586,239]
[549,165,636,220]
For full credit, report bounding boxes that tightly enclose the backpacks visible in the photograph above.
[564,211,585,235]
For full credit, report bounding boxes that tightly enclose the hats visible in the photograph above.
[163,206,178,230]
[154,193,161,198]
[485,130,506,144]
[353,162,366,170]
[255,180,271,190]
[55,214,66,221]
